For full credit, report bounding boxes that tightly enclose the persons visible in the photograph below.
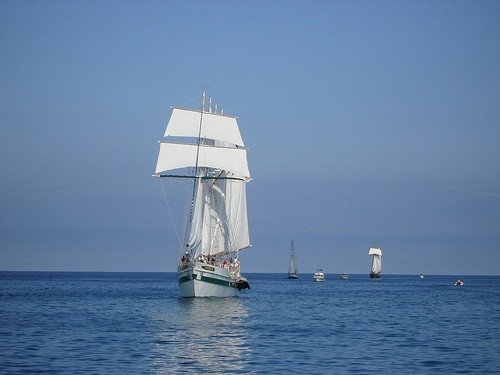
[180,252,240,271]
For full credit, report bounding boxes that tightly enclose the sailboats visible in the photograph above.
[289,239,299,279]
[368,240,382,278]
[151,90,251,298]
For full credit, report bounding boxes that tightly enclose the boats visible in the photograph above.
[455,279,463,287]
[339,273,347,279]
[313,270,325,283]
[419,274,424,279]
